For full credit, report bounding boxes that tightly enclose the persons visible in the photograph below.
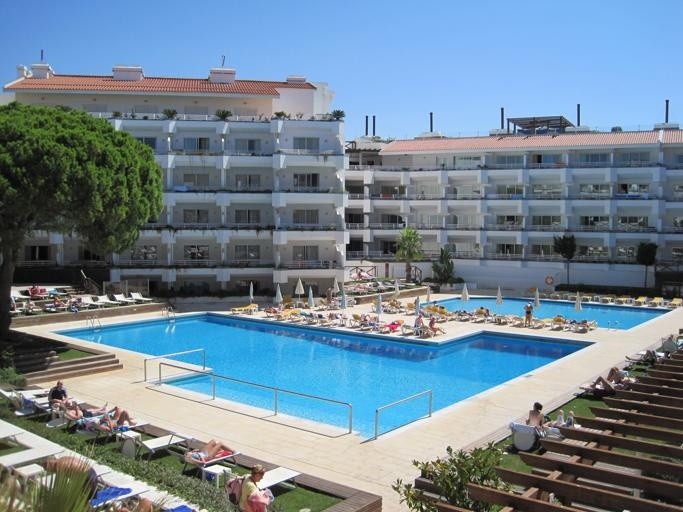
[439,305,448,315]
[31,284,39,295]
[356,269,368,278]
[333,294,339,307]
[414,313,424,329]
[527,402,559,430]
[462,310,476,316]
[57,400,110,420]
[523,302,534,327]
[40,455,98,502]
[591,332,683,394]
[82,405,138,432]
[565,410,575,428]
[10,295,82,313]
[476,306,489,317]
[179,439,236,464]
[326,286,334,309]
[327,312,381,329]
[428,316,447,334]
[555,316,576,324]
[555,410,565,424]
[583,320,596,327]
[353,284,364,296]
[46,380,70,409]
[237,463,269,512]
[108,499,154,512]
[269,305,281,313]
[413,299,423,310]
[390,299,401,309]
[433,305,440,313]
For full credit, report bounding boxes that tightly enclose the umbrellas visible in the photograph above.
[307,286,315,312]
[274,283,283,308]
[534,287,540,313]
[495,285,504,307]
[394,280,401,298]
[374,293,381,323]
[416,295,421,315]
[574,291,583,321]
[333,276,340,297]
[340,289,347,317]
[249,280,255,305]
[461,282,470,309]
[294,277,305,302]
[426,284,431,305]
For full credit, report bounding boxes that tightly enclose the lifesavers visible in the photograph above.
[545,276,554,285]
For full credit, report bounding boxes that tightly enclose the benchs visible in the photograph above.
[504,344,683,457]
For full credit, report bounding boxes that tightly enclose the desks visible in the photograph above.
[201,464,231,488]
[14,463,45,485]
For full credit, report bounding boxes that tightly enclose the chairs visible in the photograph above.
[524,287,682,309]
[30,465,111,489]
[0,288,153,327]
[344,280,415,295]
[124,434,189,459]
[2,444,65,473]
[1,426,25,444]
[224,465,301,491]
[1,386,151,443]
[436,305,597,333]
[231,296,448,342]
[88,482,150,509]
[167,447,240,476]
